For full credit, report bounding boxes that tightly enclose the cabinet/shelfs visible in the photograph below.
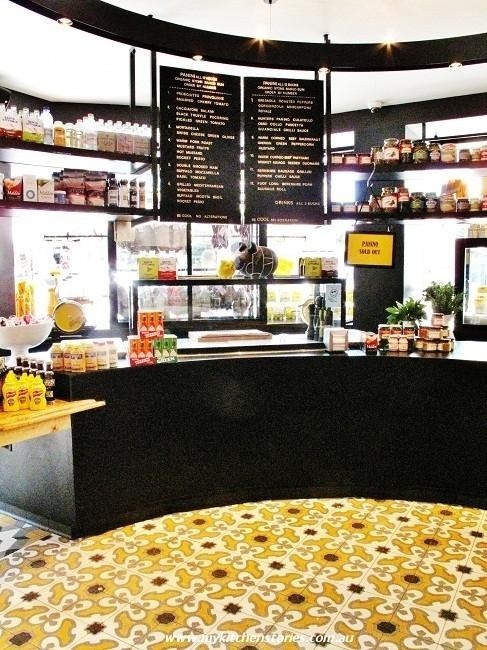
[127,274,347,355]
[0,135,160,217]
[323,158,487,221]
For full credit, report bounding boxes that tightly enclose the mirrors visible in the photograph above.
[52,299,87,335]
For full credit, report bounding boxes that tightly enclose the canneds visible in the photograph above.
[332,138,487,166]
[331,186,487,214]
[377,313,454,354]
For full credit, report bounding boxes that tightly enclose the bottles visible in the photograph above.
[52,340,118,372]
[2,355,54,411]
[109,174,145,208]
[0,103,149,156]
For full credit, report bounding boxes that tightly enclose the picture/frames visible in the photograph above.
[344,230,397,269]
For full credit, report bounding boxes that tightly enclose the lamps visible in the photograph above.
[57,0,76,26]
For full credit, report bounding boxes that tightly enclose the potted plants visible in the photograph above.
[421,281,464,326]
[385,297,428,336]
